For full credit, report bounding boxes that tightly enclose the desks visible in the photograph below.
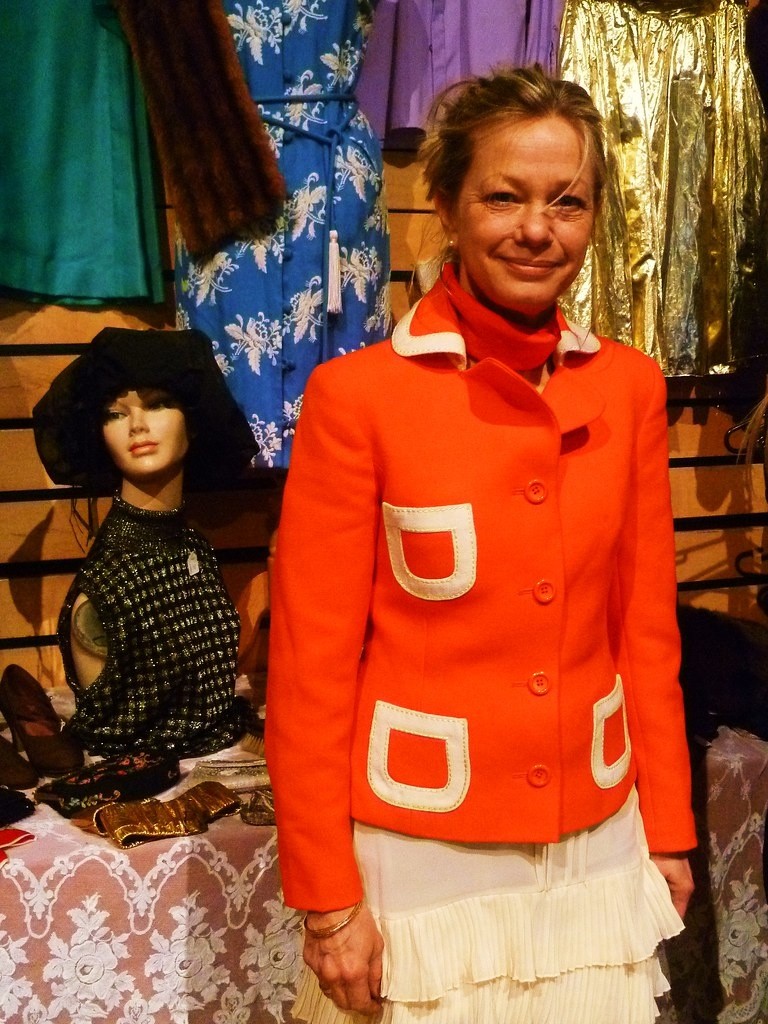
[0,726,768,1024]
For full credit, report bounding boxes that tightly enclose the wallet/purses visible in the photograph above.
[34,751,181,819]
[187,757,271,793]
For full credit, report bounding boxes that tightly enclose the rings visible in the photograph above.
[319,985,331,995]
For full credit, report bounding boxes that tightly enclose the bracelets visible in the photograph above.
[303,903,362,939]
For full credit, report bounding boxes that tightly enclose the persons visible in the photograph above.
[264,64,698,1024]
[29,328,260,767]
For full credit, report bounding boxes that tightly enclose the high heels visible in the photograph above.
[0,664,83,777]
[0,734,39,789]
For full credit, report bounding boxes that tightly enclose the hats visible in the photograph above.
[32,328,260,488]
